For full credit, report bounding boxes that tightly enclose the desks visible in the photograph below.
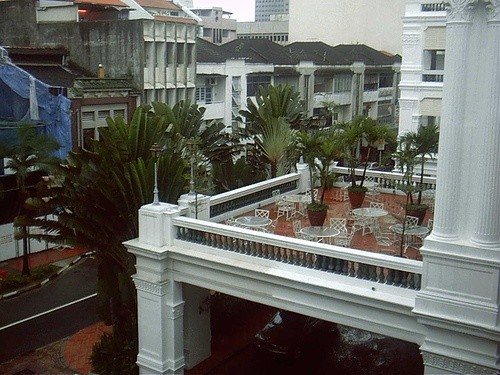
[301,225,341,243]
[281,194,310,217]
[235,215,273,232]
[388,222,431,254]
[350,207,389,237]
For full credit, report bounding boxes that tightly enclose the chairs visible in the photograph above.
[292,219,306,239]
[427,218,433,230]
[303,187,319,218]
[343,202,356,228]
[404,215,420,242]
[272,188,284,213]
[329,217,348,246]
[254,208,270,218]
[369,189,384,209]
[265,217,279,233]
[224,215,235,225]
[370,224,398,254]
[276,200,296,223]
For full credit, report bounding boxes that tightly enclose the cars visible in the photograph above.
[252,308,337,363]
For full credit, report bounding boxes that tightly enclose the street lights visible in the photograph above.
[42,196,52,249]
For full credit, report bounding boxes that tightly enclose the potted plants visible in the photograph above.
[292,104,439,229]
